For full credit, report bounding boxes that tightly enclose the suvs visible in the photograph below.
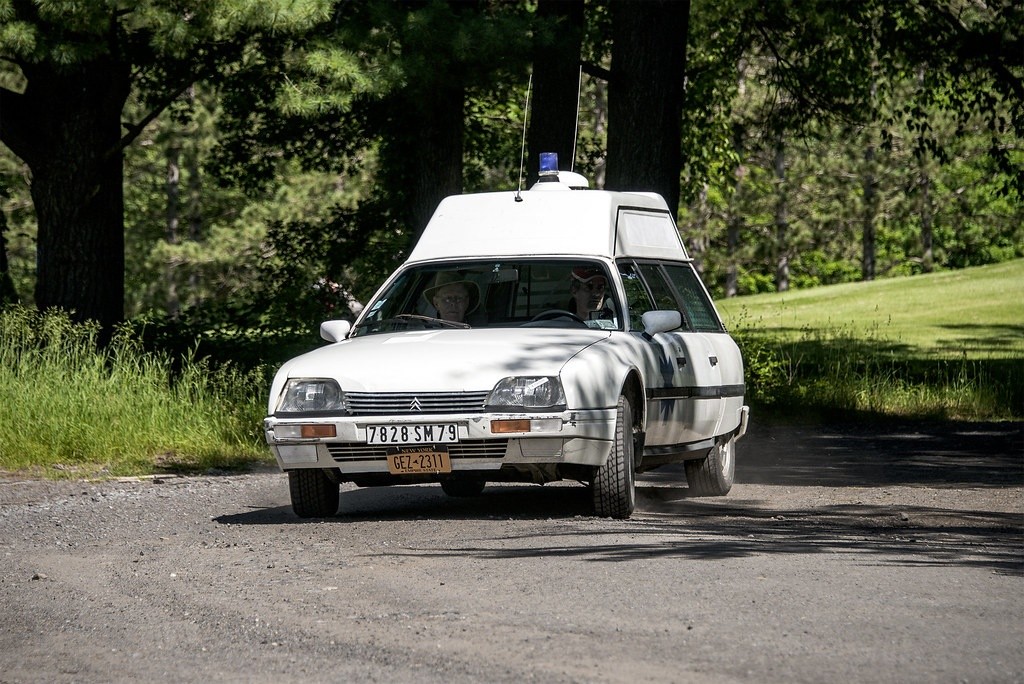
[261,173,754,523]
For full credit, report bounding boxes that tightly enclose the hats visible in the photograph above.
[423,271,483,316]
[570,265,608,288]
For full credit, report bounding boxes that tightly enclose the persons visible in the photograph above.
[570,262,609,321]
[423,272,482,330]
